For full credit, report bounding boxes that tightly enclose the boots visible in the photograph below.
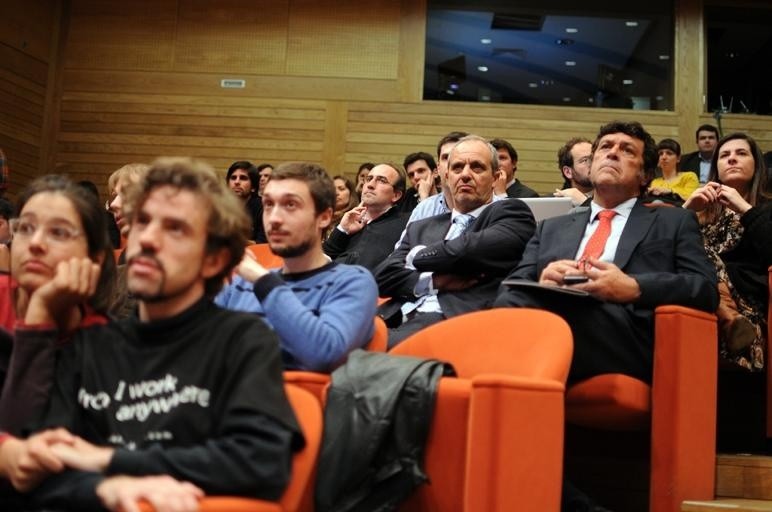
[716,283,756,350]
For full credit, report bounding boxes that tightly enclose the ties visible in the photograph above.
[577,210,616,270]
[401,215,474,316]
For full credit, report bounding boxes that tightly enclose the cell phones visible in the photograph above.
[563,276,588,285]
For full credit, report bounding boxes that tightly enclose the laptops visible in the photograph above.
[514,197,573,229]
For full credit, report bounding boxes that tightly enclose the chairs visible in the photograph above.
[1,198,771,512]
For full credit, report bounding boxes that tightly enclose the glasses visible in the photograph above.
[9,219,80,245]
